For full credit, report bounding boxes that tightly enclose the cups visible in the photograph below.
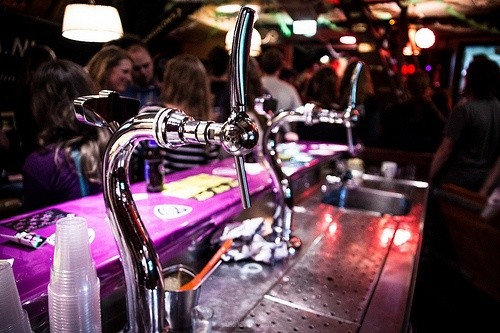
[164,264,202,333]
[47,216,102,333]
[0,261,33,333]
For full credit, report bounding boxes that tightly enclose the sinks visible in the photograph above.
[320,181,410,216]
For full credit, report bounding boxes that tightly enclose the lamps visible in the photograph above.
[62,0,125,43]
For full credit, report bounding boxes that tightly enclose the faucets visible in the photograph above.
[219,7,259,209]
[344,62,363,153]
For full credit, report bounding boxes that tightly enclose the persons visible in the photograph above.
[16,43,500,205]
